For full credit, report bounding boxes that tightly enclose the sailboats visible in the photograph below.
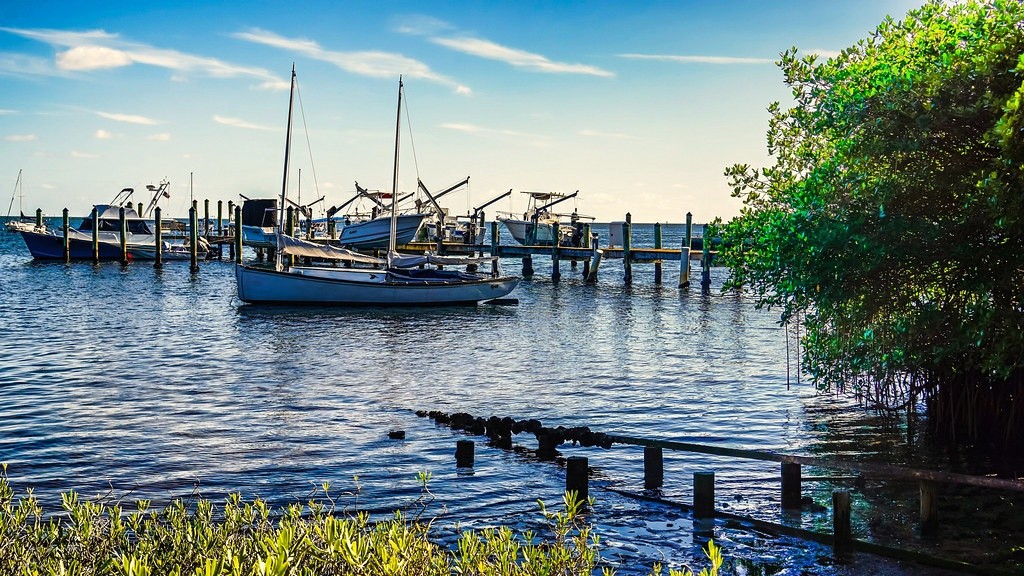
[237,62,522,303]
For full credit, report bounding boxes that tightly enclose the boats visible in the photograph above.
[145,218,203,235]
[20,176,207,260]
[496,190,596,247]
[5,170,47,232]
[241,167,513,249]
